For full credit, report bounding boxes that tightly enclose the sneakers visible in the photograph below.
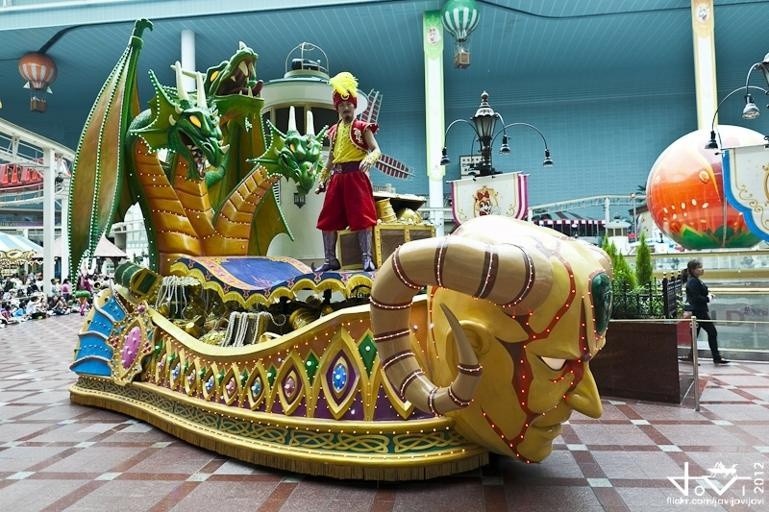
[714,359,730,365]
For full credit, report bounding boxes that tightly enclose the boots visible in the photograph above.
[311,228,341,273]
[357,227,376,272]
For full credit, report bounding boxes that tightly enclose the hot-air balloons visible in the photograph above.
[439,0,483,70]
[17,51,56,113]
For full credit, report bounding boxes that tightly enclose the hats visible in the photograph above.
[331,71,358,109]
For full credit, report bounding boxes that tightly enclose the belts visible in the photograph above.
[332,162,360,171]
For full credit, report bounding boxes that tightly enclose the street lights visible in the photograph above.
[703,51,769,154]
[438,88,557,174]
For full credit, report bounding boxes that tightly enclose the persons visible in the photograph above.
[311,73,381,272]
[0,274,112,325]
[682,260,731,364]
